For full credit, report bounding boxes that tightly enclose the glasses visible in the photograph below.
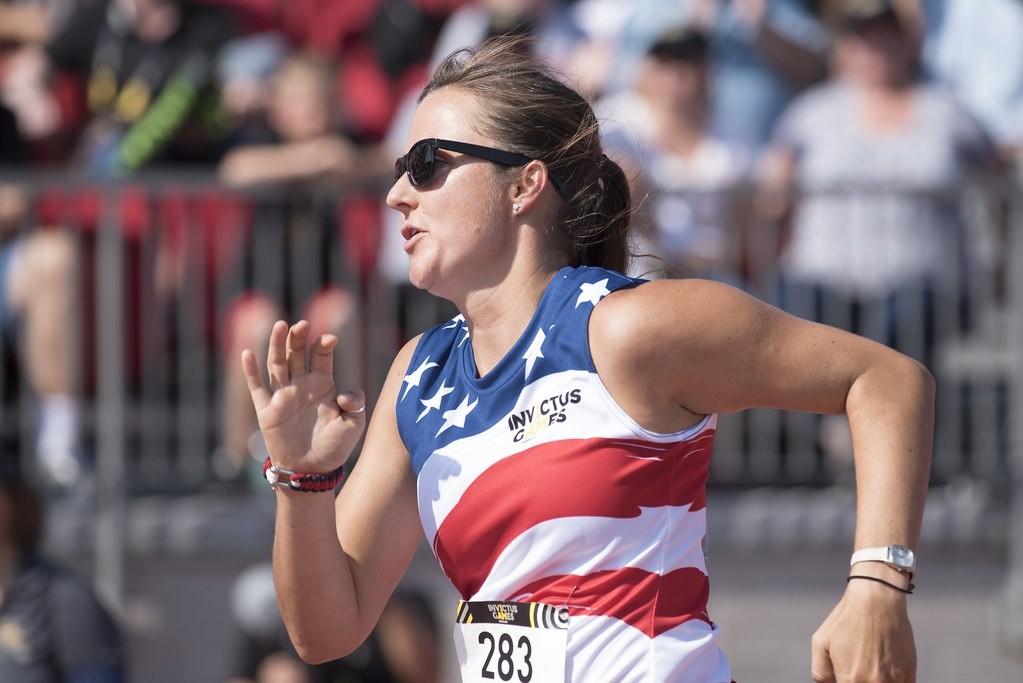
[394,137,571,198]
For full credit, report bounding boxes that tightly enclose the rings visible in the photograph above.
[347,405,365,414]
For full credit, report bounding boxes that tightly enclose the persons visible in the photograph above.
[242,24,939,683]
[1,470,448,683]
[0,0,1021,492]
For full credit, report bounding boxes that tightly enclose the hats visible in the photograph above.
[646,27,703,62]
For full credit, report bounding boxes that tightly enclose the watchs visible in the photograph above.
[847,545,918,576]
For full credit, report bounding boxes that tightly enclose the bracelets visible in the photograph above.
[847,573,918,595]
[261,459,347,494]
